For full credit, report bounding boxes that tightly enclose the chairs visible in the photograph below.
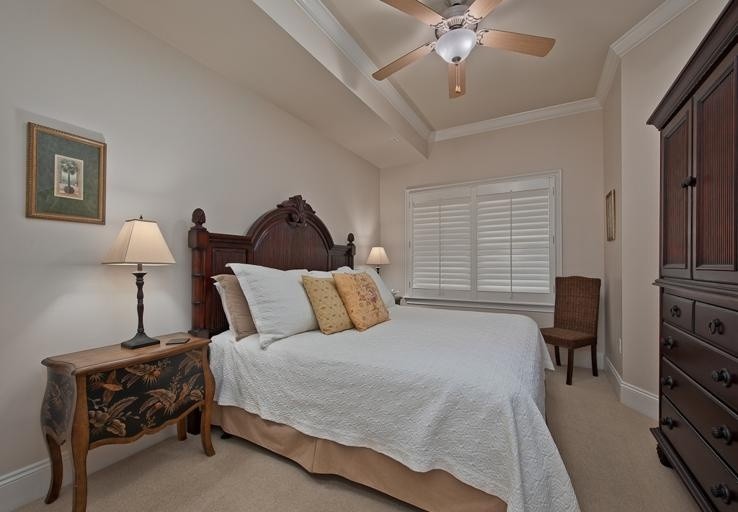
[540,276,601,385]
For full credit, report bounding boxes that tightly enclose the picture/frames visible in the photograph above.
[26,122,107,225]
[605,189,615,242]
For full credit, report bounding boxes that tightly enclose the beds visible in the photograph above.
[189,194,582,512]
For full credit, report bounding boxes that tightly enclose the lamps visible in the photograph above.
[101,215,177,350]
[434,28,477,65]
[366,247,391,274]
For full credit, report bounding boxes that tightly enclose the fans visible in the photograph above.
[372,0,556,100]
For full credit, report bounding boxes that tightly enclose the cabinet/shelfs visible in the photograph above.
[644,0,738,512]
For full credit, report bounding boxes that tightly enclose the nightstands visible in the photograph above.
[395,296,403,305]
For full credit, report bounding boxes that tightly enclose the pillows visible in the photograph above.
[212,282,236,344]
[210,274,257,341]
[225,263,319,348]
[331,271,391,332]
[310,266,354,274]
[357,266,395,310]
[302,275,355,334]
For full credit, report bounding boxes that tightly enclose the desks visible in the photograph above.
[39,331,216,511]
